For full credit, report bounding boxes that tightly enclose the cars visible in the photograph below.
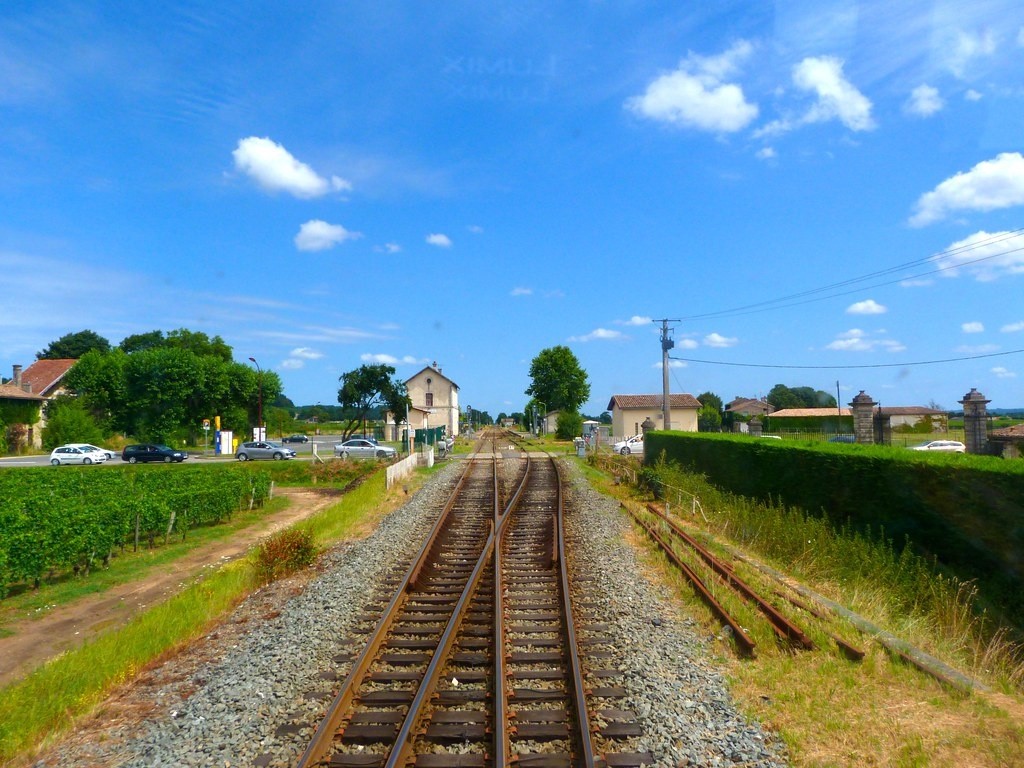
[612,433,644,455]
[63,442,116,461]
[121,443,188,464]
[334,438,397,459]
[351,433,378,445]
[50,445,107,466]
[827,436,855,443]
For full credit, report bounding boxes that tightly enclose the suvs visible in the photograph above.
[904,440,966,454]
[282,435,308,444]
[234,441,296,462]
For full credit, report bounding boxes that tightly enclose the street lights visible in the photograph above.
[538,402,547,415]
[248,357,262,441]
